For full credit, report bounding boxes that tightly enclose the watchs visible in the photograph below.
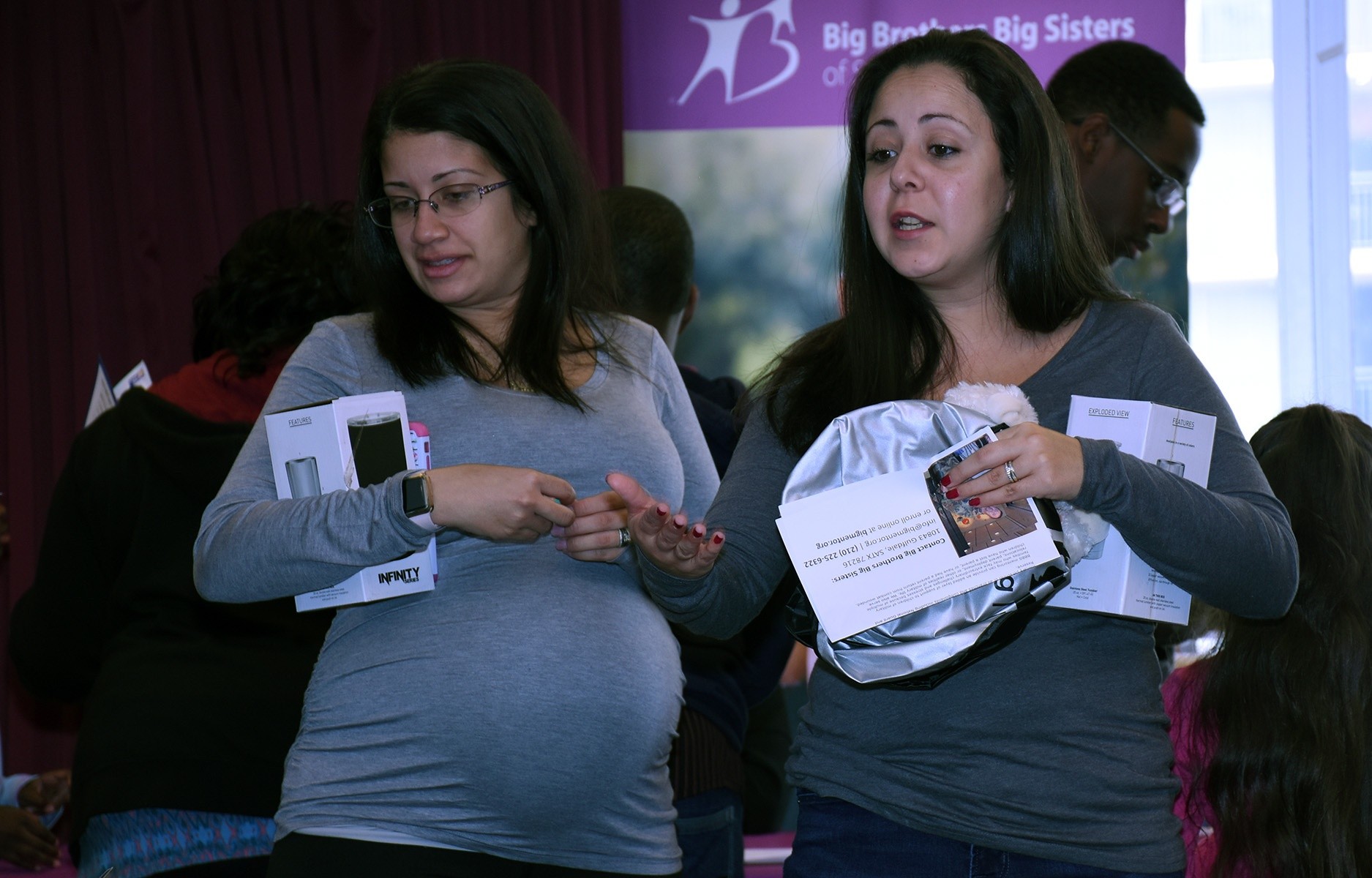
[402,470,441,532]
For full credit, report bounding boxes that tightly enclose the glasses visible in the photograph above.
[1107,121,1186,217]
[364,178,515,228]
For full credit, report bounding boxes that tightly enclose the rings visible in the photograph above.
[1005,460,1018,483]
[618,527,630,548]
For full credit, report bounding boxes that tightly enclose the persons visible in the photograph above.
[0,730,74,869]
[8,198,400,878]
[193,59,722,878]
[1032,39,1203,273]
[567,186,814,878]
[606,30,1299,878]
[1161,406,1372,878]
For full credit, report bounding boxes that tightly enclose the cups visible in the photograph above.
[347,412,408,488]
